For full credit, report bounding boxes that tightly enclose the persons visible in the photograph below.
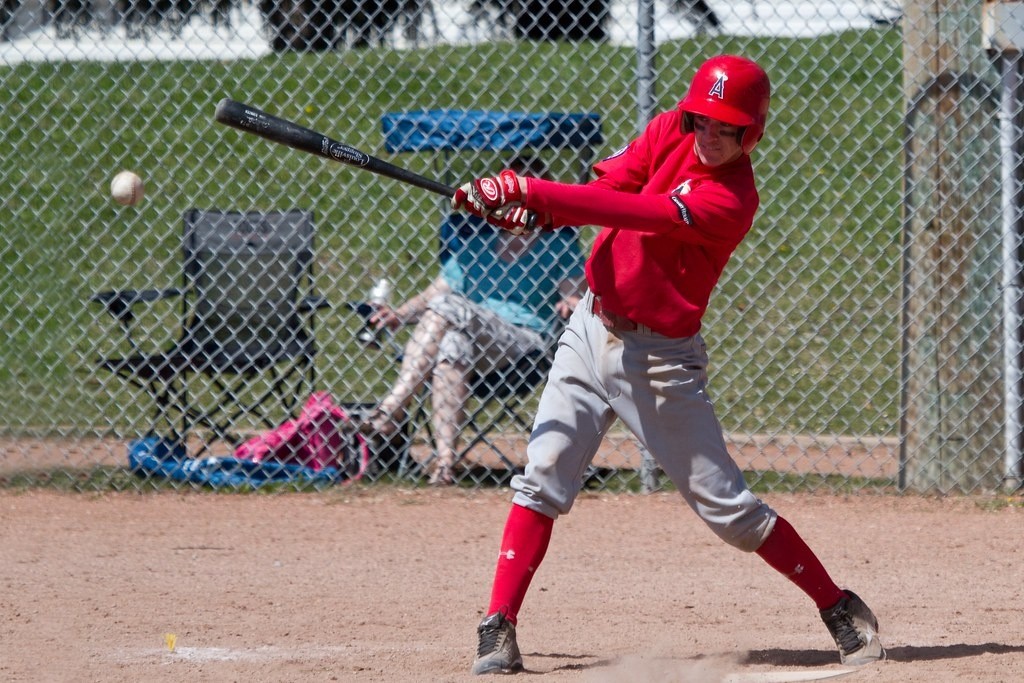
[360,151,587,485]
[450,53,886,673]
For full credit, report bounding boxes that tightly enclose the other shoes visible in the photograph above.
[359,408,397,435]
[427,465,454,488]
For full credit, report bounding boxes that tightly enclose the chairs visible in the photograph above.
[381,108,608,490]
[93,208,331,458]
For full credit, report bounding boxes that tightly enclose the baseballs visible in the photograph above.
[109,170,145,204]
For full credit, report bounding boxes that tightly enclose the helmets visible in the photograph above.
[678,53,771,155]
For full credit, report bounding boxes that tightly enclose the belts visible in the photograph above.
[591,297,637,331]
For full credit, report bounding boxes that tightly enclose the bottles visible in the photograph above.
[358,279,393,350]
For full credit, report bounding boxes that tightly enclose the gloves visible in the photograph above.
[449,169,522,219]
[484,200,533,236]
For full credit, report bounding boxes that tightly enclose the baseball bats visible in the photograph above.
[212,98,537,231]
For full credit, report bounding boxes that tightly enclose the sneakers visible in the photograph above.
[473,605,522,676]
[819,589,886,668]
